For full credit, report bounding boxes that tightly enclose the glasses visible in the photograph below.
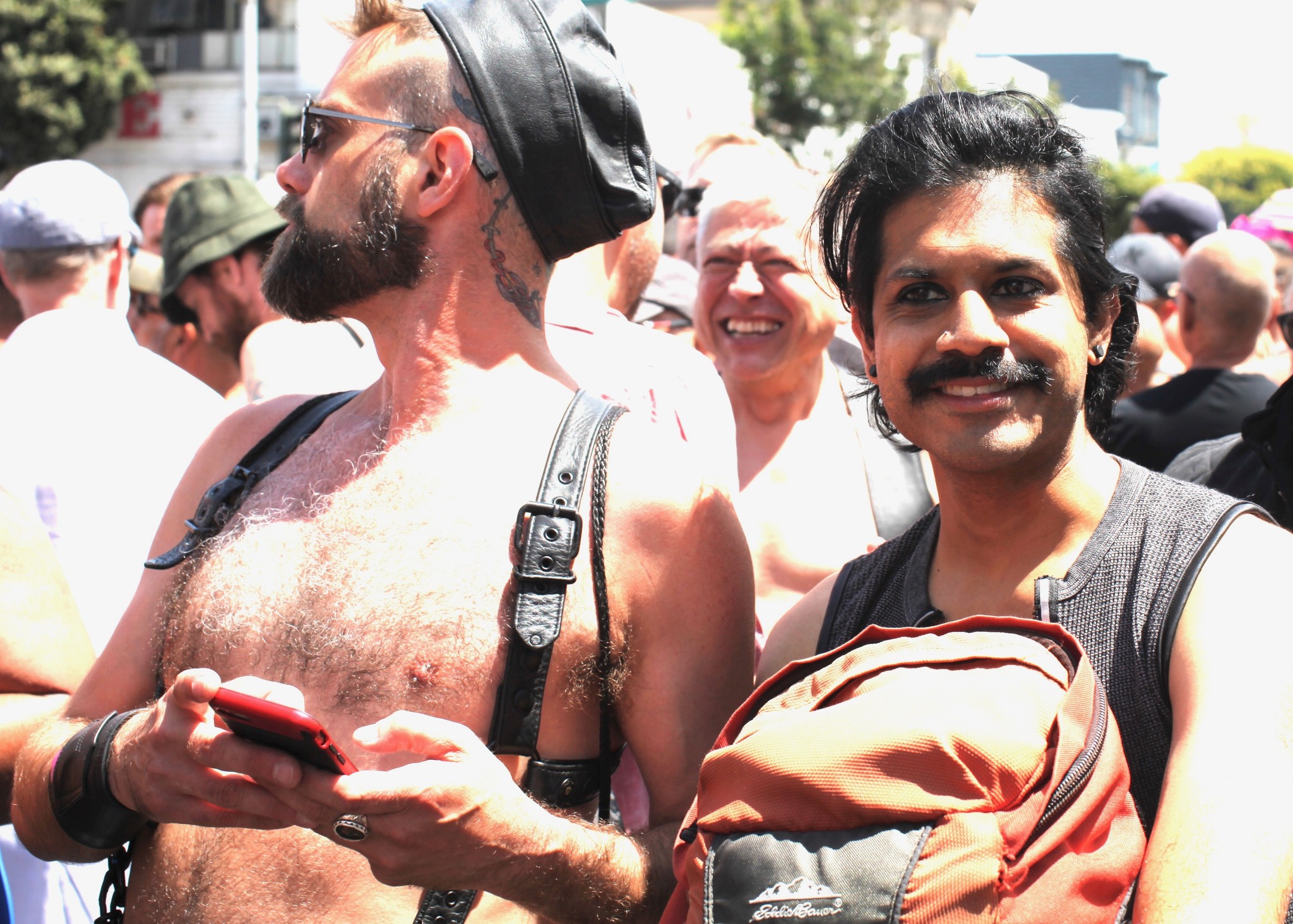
[300,103,498,182]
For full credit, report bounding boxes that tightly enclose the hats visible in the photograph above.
[1132,180,1228,246]
[422,0,653,260]
[0,160,128,251]
[1108,232,1182,300]
[160,172,289,327]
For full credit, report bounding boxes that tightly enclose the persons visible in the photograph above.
[16,5,758,924]
[754,93,1293,924]
[0,1,1293,924]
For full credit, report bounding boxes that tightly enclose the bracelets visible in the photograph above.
[45,708,153,847]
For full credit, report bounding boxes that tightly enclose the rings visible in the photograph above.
[331,808,373,842]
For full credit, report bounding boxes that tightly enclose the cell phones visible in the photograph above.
[208,688,359,777]
